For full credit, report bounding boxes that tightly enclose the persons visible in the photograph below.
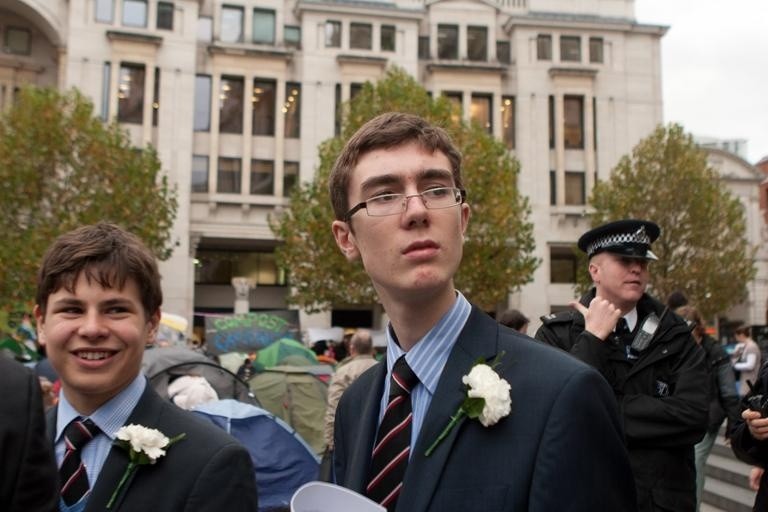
[327,113,636,512]
[530,220,709,512]
[328,329,380,453]
[0,348,62,511]
[32,223,258,512]
[497,309,529,335]
[668,289,768,512]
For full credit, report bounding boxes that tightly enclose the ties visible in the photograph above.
[361,354,422,511]
[56,417,100,507]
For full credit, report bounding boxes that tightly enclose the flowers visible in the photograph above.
[419,346,516,459]
[103,421,190,510]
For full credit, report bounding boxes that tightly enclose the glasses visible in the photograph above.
[342,184,463,222]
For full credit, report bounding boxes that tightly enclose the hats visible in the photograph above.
[576,219,661,265]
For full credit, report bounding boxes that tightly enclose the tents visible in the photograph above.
[141,310,336,512]
